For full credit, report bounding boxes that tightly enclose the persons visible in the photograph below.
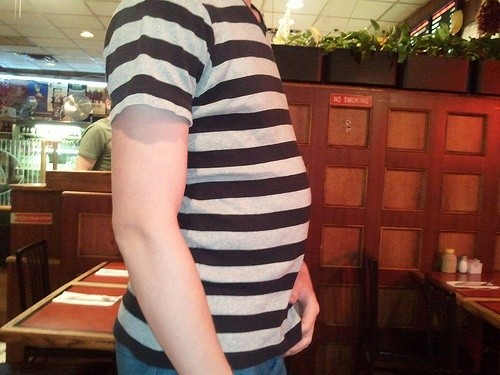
[1,80,103,114]
[103,1,320,375]
[76,84,113,171]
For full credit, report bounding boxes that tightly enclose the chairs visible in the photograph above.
[14,237,52,315]
[360,247,469,375]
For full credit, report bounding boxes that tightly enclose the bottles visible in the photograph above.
[441,248,457,273]
[458,255,469,273]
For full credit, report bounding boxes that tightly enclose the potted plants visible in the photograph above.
[264,14,500,99]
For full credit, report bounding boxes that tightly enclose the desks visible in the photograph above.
[0,260,136,375]
[427,267,500,375]
